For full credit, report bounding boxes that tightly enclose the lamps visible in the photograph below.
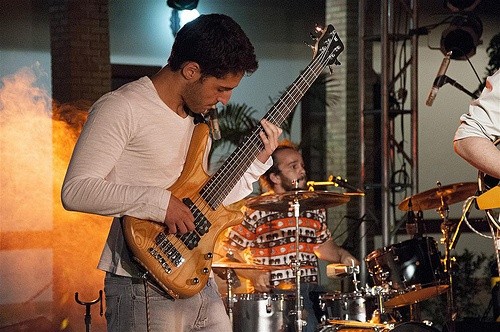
[441,11,484,60]
[167,0,199,11]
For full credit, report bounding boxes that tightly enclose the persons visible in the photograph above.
[62,13,283,332]
[221,145,359,332]
[453,66,500,181]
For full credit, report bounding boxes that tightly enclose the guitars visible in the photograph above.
[118,22,345,301]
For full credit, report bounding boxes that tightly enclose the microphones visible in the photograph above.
[426,50,453,106]
[406,200,417,236]
[448,199,475,251]
[209,104,221,141]
[328,174,363,192]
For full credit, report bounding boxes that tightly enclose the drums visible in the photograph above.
[364,234,450,309]
[316,317,435,332]
[309,288,389,323]
[229,291,304,332]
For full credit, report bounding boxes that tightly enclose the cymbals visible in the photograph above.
[211,255,315,270]
[399,181,480,212]
[246,191,350,212]
